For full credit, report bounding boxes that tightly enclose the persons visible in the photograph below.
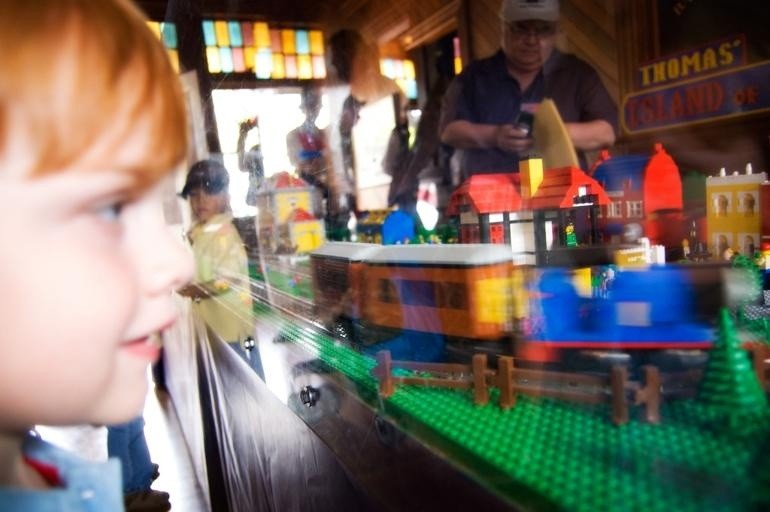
[326,24,416,210]
[288,80,341,216]
[236,118,298,345]
[95,420,176,512]
[0,0,198,512]
[176,154,255,344]
[437,0,628,179]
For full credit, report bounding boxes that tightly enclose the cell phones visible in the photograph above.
[514,111,534,135]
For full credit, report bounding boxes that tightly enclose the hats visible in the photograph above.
[182,159,229,200]
[499,0,561,24]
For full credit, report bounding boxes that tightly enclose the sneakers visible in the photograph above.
[125,488,173,512]
